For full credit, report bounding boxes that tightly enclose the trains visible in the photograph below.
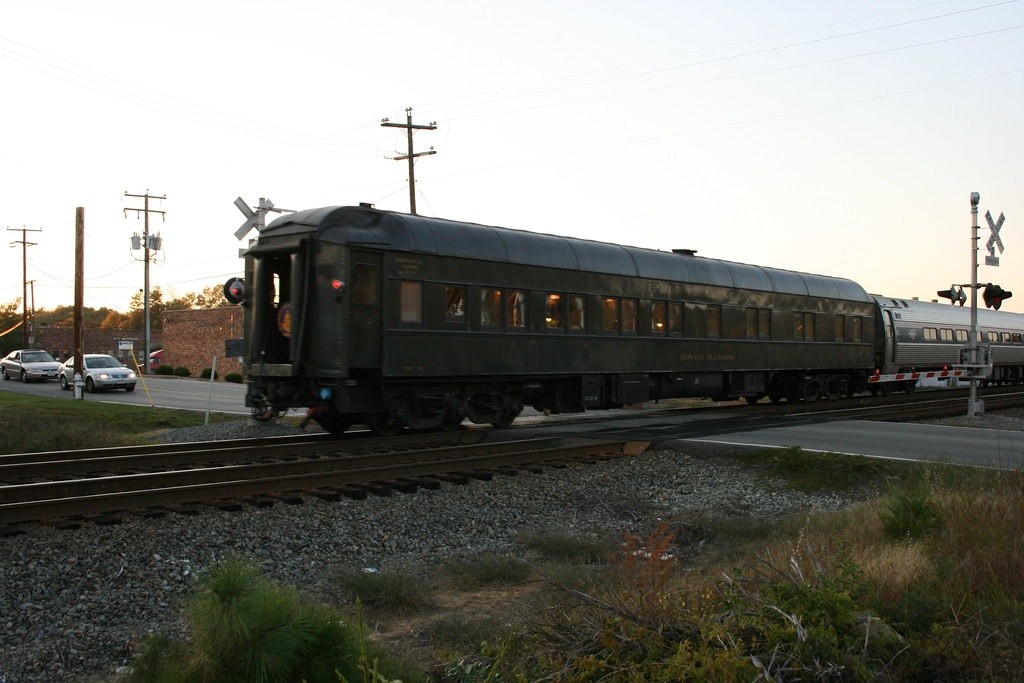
[223,202,1023,436]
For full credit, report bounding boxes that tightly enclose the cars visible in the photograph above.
[0,348,64,384]
[55,354,138,394]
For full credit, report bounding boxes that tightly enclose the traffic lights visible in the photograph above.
[982,283,1013,311]
[936,289,967,309]
